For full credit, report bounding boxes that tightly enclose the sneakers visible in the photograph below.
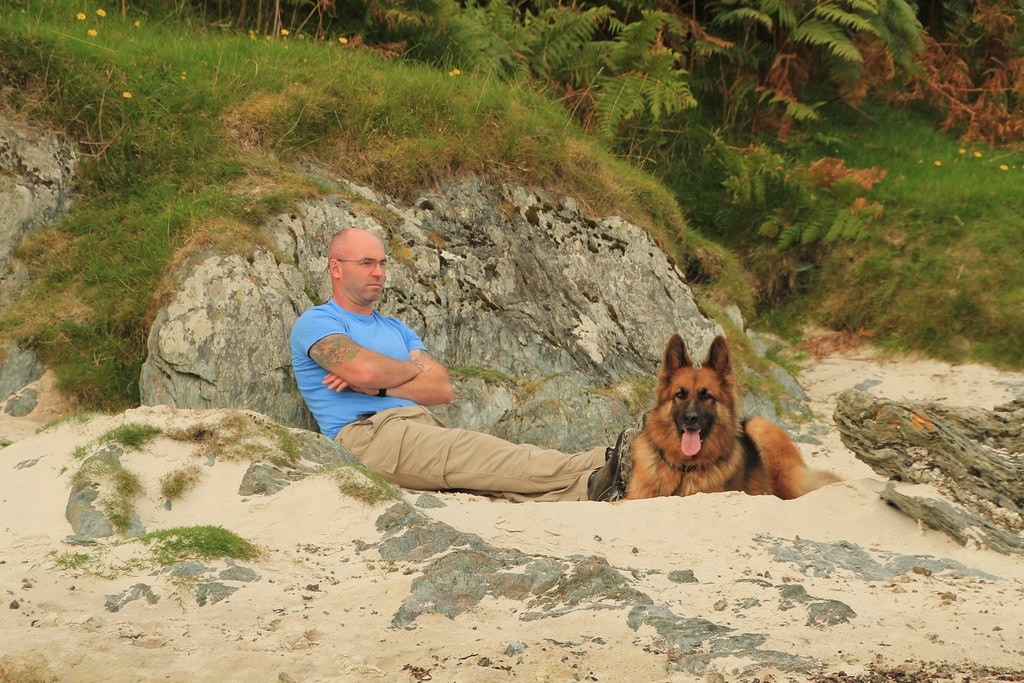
[586,428,638,502]
[606,444,615,460]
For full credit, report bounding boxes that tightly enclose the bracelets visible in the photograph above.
[378,389,387,397]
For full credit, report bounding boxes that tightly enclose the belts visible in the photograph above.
[356,412,377,421]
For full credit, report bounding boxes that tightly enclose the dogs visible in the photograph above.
[609,333,845,506]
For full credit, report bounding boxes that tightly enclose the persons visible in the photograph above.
[289,228,636,502]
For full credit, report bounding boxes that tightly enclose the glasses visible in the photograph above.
[338,258,387,270]
